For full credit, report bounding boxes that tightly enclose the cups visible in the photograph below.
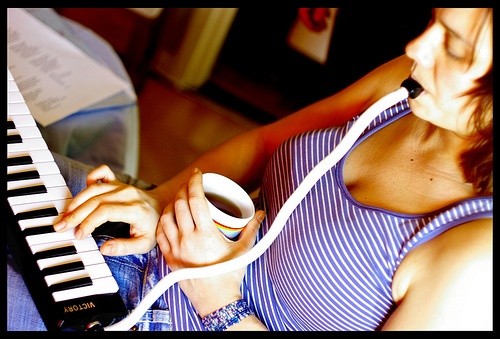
[201,172,255,241]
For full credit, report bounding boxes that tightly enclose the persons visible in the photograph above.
[8,8,492,331]
